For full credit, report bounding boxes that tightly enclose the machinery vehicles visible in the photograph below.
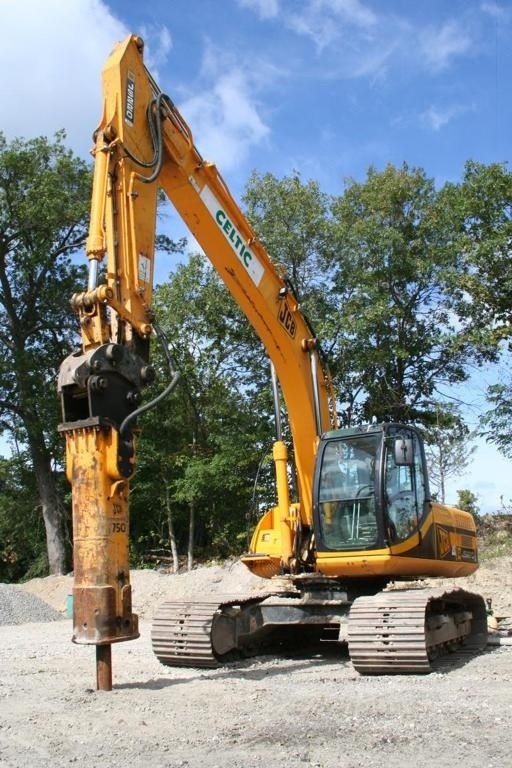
[58,32,491,692]
